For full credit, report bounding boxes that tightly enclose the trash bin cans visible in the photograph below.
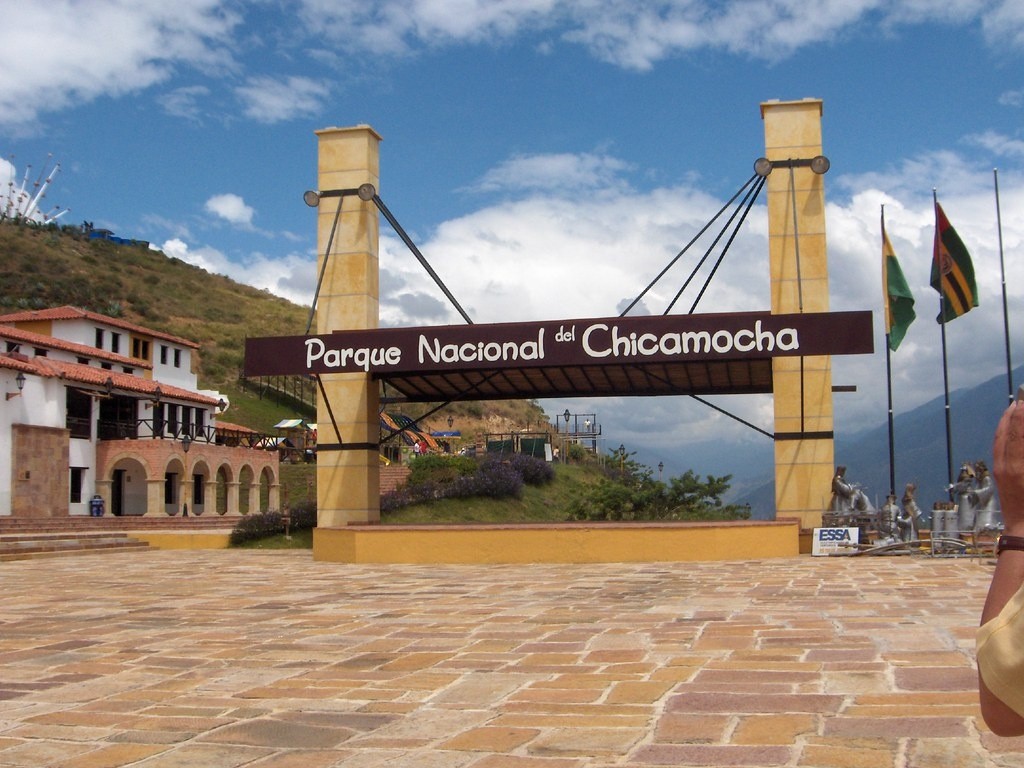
[90,499,103,516]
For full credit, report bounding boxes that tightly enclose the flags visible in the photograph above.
[929,202,979,323]
[881,228,916,351]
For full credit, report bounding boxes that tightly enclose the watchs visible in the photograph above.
[994,534,1024,557]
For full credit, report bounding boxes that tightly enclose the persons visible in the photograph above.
[312,429,317,445]
[552,446,560,462]
[832,467,868,514]
[880,484,925,547]
[442,441,450,452]
[461,448,465,454]
[414,439,427,458]
[945,459,994,536]
[584,419,591,432]
[976,385,1024,737]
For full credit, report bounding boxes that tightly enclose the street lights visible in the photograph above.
[562,409,570,457]
[303,417,308,449]
[618,445,626,475]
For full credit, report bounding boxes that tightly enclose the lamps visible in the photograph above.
[753,158,772,177]
[94,377,114,401]
[145,386,163,409]
[209,398,227,419]
[357,184,376,201]
[810,156,830,174]
[304,191,321,207]
[6,371,27,401]
